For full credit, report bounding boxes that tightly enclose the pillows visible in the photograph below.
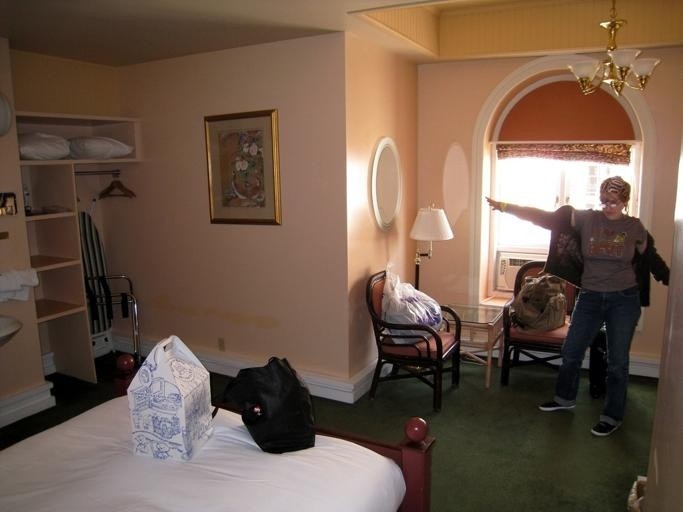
[66,136,135,160]
[17,131,71,161]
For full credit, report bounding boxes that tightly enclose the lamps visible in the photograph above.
[409,203,454,290]
[567,1,662,98]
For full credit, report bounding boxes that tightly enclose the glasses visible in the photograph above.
[599,203,617,209]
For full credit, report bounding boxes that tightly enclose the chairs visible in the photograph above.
[366,271,462,413]
[501,262,580,387]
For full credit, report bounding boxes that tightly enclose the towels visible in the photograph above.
[1,268,40,303]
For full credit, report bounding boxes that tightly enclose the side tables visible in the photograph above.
[440,302,511,390]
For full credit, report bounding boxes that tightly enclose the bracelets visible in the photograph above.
[501,202,507,211]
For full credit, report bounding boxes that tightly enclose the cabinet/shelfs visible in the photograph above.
[0,37,23,403]
[15,110,144,384]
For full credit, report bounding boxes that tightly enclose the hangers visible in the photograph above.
[98,169,135,198]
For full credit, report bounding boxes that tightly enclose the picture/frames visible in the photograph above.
[204,109,283,226]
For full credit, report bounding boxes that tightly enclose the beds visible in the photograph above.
[0,354,437,512]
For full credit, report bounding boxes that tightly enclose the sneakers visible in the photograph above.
[591,421,625,436]
[538,400,576,412]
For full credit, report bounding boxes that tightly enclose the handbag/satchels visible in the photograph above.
[127,335,215,464]
[232,356,315,454]
[509,274,568,330]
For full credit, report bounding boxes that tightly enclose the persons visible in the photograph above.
[486,176,671,437]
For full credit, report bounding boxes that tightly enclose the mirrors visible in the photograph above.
[370,135,402,233]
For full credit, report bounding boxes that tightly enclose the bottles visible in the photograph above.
[22,184,30,208]
